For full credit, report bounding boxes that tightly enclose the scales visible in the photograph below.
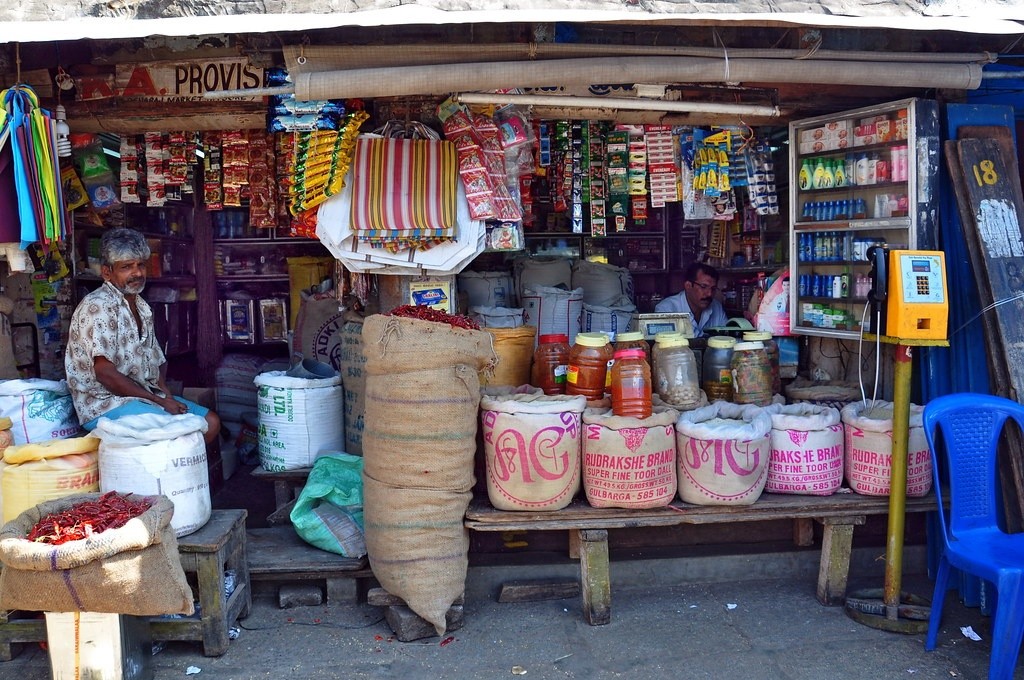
[631,312,694,341]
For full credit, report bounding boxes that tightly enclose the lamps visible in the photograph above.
[452,92,780,117]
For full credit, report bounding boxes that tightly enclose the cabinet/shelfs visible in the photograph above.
[212,201,321,346]
[789,97,938,344]
[524,204,671,275]
[678,190,789,315]
[68,158,197,356]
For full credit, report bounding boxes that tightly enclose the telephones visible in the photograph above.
[867,246,948,338]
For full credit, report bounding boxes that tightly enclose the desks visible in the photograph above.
[0,509,253,656]
[465,484,951,627]
[251,465,312,511]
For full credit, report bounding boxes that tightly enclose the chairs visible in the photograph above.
[922,391,1024,680]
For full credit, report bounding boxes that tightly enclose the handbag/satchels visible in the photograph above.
[314,118,486,277]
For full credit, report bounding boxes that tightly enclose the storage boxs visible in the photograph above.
[182,386,216,409]
[802,302,870,331]
[800,109,907,154]
[745,271,799,366]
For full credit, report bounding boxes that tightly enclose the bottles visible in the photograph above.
[536,238,567,253]
[535,330,782,419]
[721,277,759,311]
[797,145,914,299]
[732,244,754,267]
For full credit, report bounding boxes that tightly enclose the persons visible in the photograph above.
[654,262,728,339]
[769,277,790,313]
[64,227,221,445]
[714,204,726,213]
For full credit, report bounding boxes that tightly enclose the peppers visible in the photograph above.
[26,488,154,544]
[380,303,481,330]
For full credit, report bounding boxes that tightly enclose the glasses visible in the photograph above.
[690,281,721,293]
